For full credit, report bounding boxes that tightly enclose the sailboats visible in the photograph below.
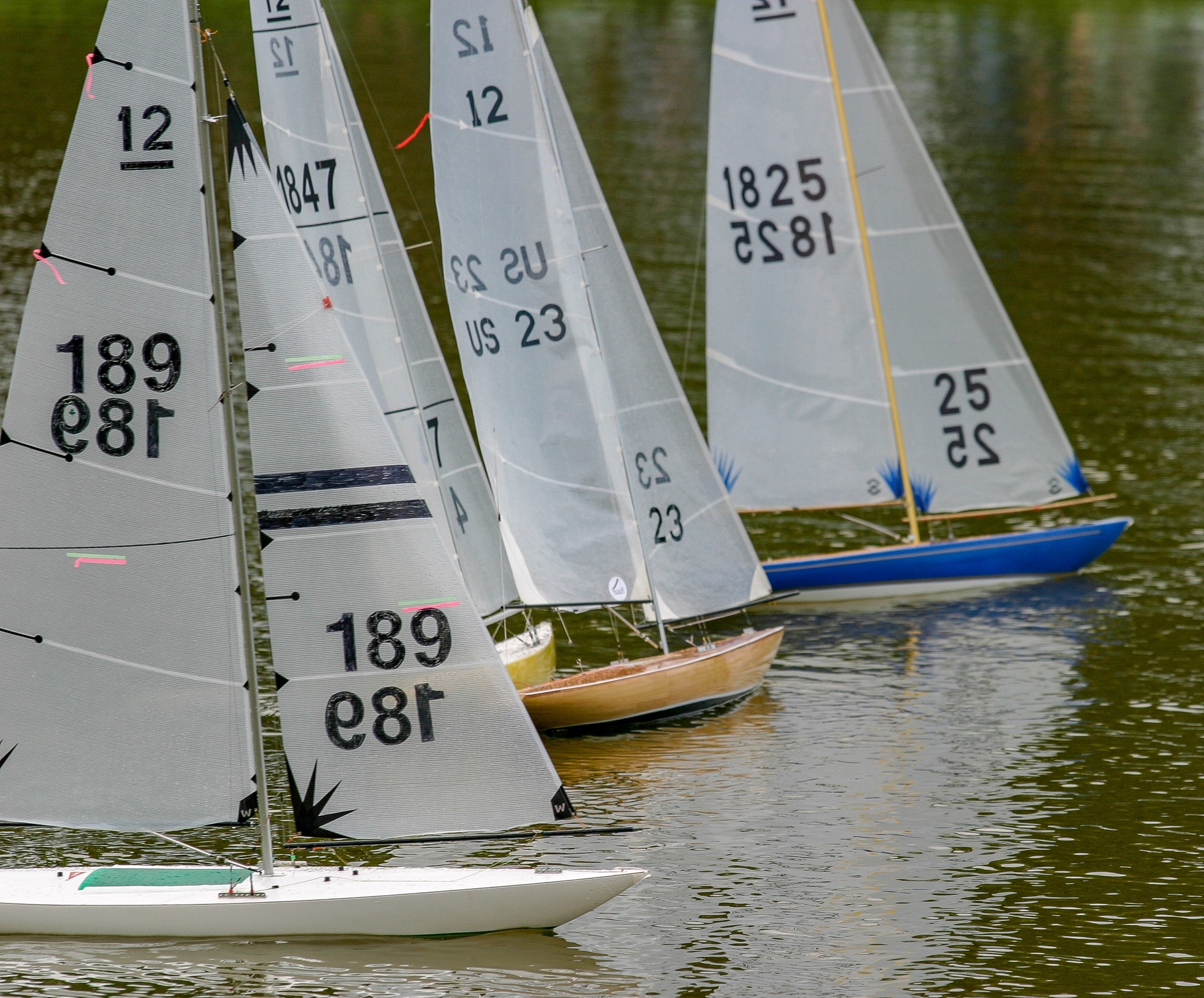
[1,0,1136,938]
